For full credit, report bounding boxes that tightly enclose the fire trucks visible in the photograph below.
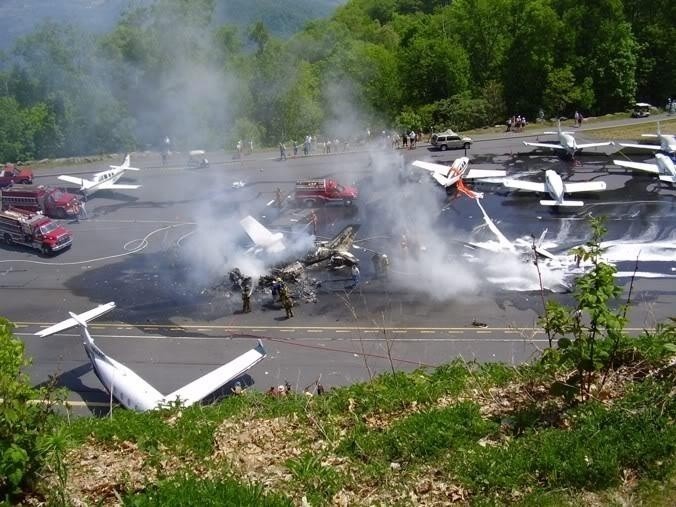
[295,177,357,207]
[0,161,83,256]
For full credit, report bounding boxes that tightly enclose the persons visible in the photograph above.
[71,202,82,223]
[667,95,672,105]
[670,99,676,105]
[279,135,312,160]
[308,209,318,233]
[399,233,408,258]
[371,251,381,277]
[77,200,89,219]
[507,115,526,132]
[574,111,584,128]
[274,184,283,208]
[159,135,169,166]
[226,263,300,317]
[349,261,361,287]
[379,254,392,276]
[323,130,416,152]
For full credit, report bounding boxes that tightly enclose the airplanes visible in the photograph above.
[202,219,368,284]
[58,155,142,198]
[498,167,605,211]
[609,152,676,190]
[409,154,503,199]
[33,299,268,405]
[622,124,676,152]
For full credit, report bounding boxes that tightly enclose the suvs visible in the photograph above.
[632,103,651,118]
[433,132,475,151]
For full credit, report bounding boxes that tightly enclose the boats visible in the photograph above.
[523,120,610,155]
